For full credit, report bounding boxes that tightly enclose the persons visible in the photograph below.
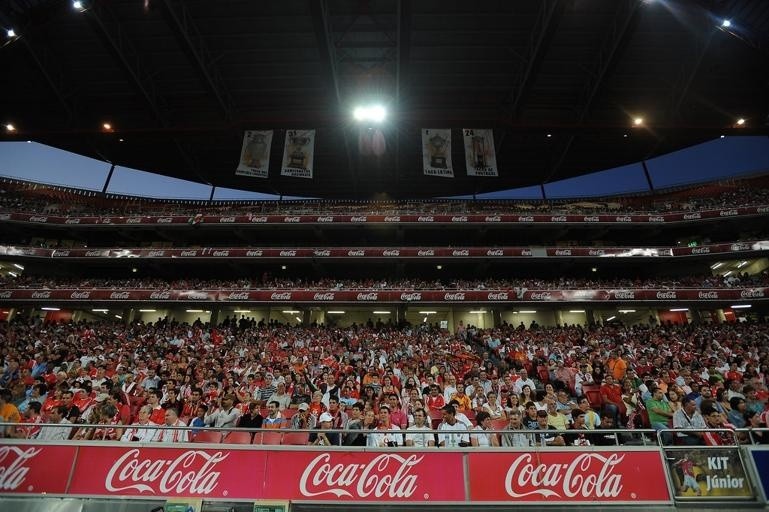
[1,185,768,216]
[1,312,769,449]
[1,236,769,250]
[671,453,706,496]
[1,270,769,292]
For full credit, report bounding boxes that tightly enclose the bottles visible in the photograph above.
[541,436,546,448]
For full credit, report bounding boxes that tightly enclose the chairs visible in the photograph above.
[254,432,283,445]
[345,409,364,419]
[583,385,602,408]
[196,431,223,445]
[283,432,309,445]
[639,409,658,438]
[428,410,509,443]
[224,431,254,443]
[669,418,687,444]
[536,365,549,382]
[259,400,299,420]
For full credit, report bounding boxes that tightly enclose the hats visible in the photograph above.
[1,327,768,427]
